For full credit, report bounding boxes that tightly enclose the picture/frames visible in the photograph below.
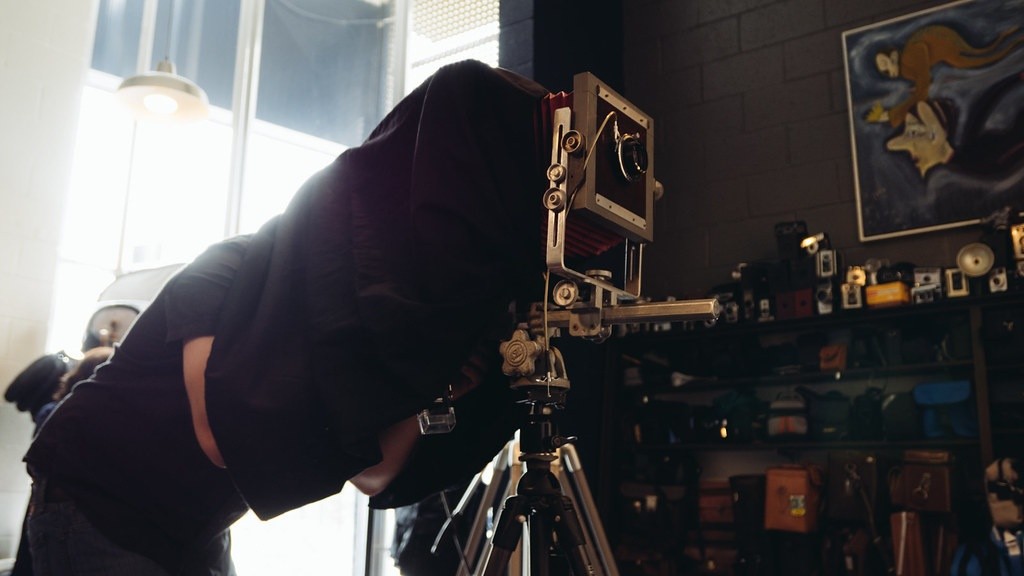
[841,0,1024,243]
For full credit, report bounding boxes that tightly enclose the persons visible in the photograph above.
[9,58,560,576]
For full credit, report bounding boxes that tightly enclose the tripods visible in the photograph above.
[452,322,618,576]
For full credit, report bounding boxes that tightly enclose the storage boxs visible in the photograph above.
[764,467,822,534]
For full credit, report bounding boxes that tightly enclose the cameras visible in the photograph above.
[544,75,664,303]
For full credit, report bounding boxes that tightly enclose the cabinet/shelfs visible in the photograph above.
[562,289,1024,576]
